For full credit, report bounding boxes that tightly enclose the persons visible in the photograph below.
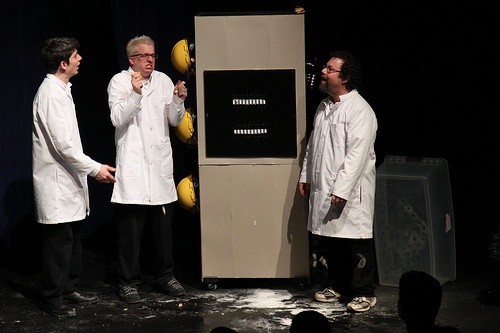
[107,35,187,303]
[299,51,380,312]
[397,270,459,333]
[32,38,116,317]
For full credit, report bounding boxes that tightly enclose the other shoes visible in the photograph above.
[115,283,141,303]
[315,287,341,303]
[38,296,77,318]
[63,292,99,304]
[159,279,185,297]
[347,296,376,312]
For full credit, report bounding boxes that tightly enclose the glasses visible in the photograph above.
[322,63,344,73]
[131,53,158,59]
